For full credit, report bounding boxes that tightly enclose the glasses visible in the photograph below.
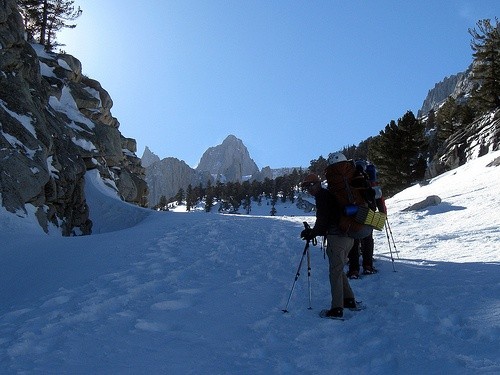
[305,182,316,188]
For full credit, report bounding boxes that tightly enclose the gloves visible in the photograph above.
[301,229,316,241]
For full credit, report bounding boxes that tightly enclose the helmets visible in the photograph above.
[326,152,353,168]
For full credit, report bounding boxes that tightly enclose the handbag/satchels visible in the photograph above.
[348,218,371,238]
[356,206,387,232]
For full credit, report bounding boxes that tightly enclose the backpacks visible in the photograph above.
[328,166,376,210]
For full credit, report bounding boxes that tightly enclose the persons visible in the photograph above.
[325,152,376,279]
[300,174,360,319]
[354,159,387,262]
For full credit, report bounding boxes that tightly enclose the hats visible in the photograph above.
[301,175,319,187]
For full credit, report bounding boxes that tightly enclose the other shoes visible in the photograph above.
[320,308,342,317]
[344,298,356,308]
[349,269,359,278]
[363,264,377,274]
